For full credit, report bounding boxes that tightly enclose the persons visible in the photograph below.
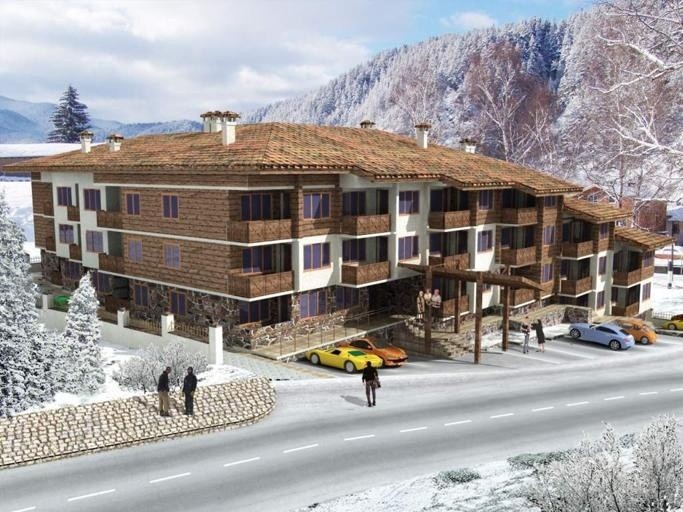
[156,366,173,417]
[530,318,545,352]
[361,360,379,408]
[521,316,533,354]
[415,290,424,324]
[180,366,197,415]
[421,288,432,319]
[429,289,441,323]
